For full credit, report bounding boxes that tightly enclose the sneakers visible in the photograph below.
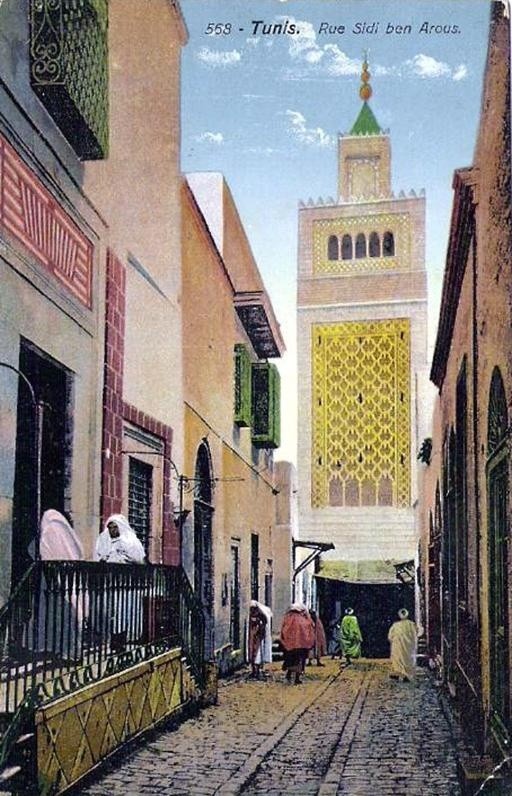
[285,675,292,683]
[403,677,410,683]
[317,662,324,666]
[390,675,399,680]
[306,663,312,666]
[295,680,302,684]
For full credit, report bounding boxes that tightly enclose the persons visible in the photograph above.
[242,598,274,683]
[304,607,327,667]
[93,514,149,658]
[339,606,364,665]
[277,601,313,687]
[18,507,90,664]
[386,607,424,681]
